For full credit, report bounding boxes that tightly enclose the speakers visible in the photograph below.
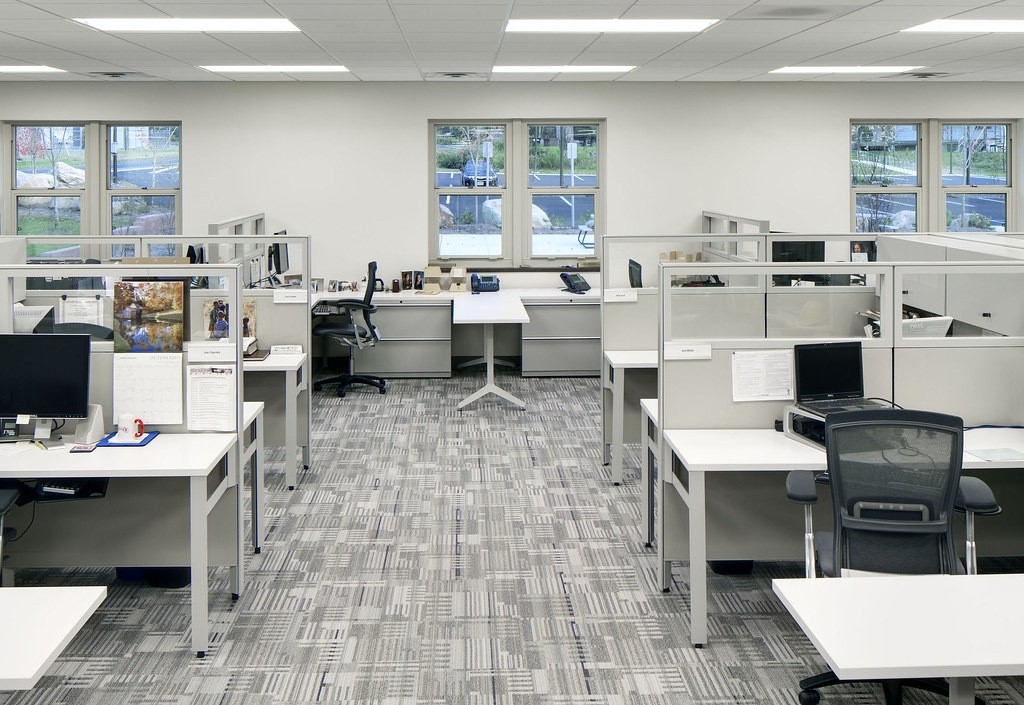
[74,404,105,445]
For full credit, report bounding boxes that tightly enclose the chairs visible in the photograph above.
[312,261,386,397]
[785,409,1002,705]
[578,220,595,249]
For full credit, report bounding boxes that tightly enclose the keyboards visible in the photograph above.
[825,460,948,491]
[41,483,80,494]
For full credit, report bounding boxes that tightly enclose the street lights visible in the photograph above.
[111,141,118,183]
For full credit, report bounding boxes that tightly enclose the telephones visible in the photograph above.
[560,271,591,292]
[471,274,500,292]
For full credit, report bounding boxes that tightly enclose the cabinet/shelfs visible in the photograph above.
[350,303,451,378]
[521,302,602,376]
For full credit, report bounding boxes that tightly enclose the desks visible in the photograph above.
[639,399,660,547]
[663,427,1024,649]
[243,401,267,554]
[603,350,658,486]
[311,288,602,414]
[242,352,308,491]
[771,573,1024,705]
[0,434,237,658]
[0,586,107,691]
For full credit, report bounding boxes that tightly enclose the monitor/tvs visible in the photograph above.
[0,331,91,452]
[13,305,56,333]
[267,230,289,274]
[770,230,828,287]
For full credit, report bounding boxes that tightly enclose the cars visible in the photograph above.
[460,160,498,186]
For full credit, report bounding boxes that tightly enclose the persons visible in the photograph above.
[402,274,412,290]
[243,316,249,337]
[208,299,229,338]
[414,274,423,289]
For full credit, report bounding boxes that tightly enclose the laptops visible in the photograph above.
[794,341,891,418]
